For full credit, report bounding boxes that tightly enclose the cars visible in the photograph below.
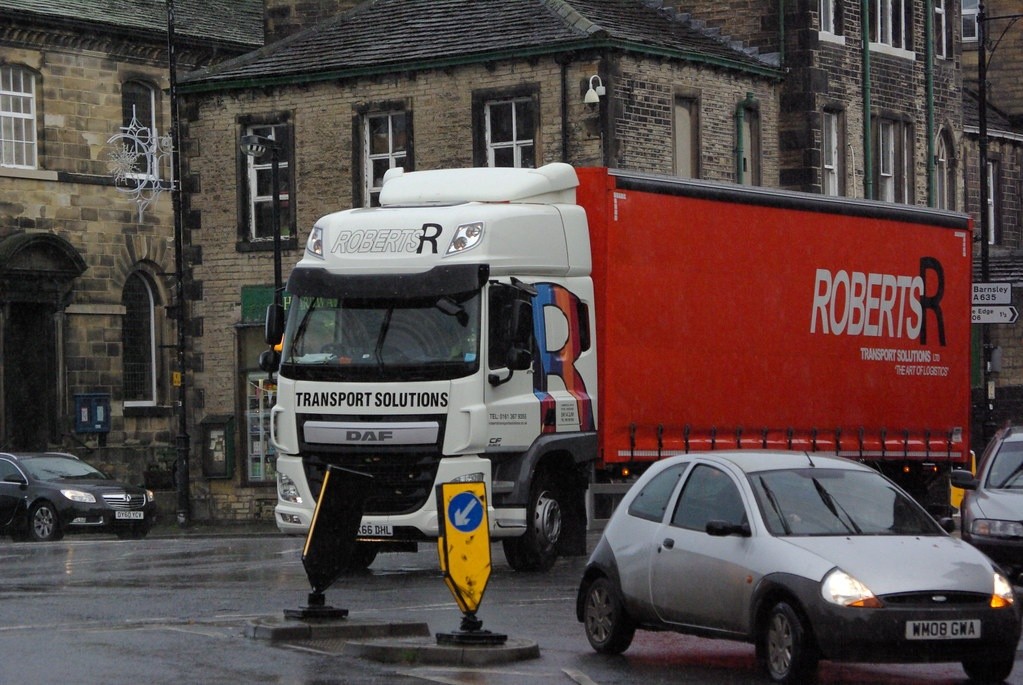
[576,449,1021,685]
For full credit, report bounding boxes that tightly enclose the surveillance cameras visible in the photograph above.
[584,89,600,109]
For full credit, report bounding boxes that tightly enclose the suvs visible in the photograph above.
[950,421,1023,573]
[0,450,156,542]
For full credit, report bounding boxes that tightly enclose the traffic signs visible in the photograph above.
[971,306,1018,323]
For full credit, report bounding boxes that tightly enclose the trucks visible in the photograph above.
[260,163,973,582]
